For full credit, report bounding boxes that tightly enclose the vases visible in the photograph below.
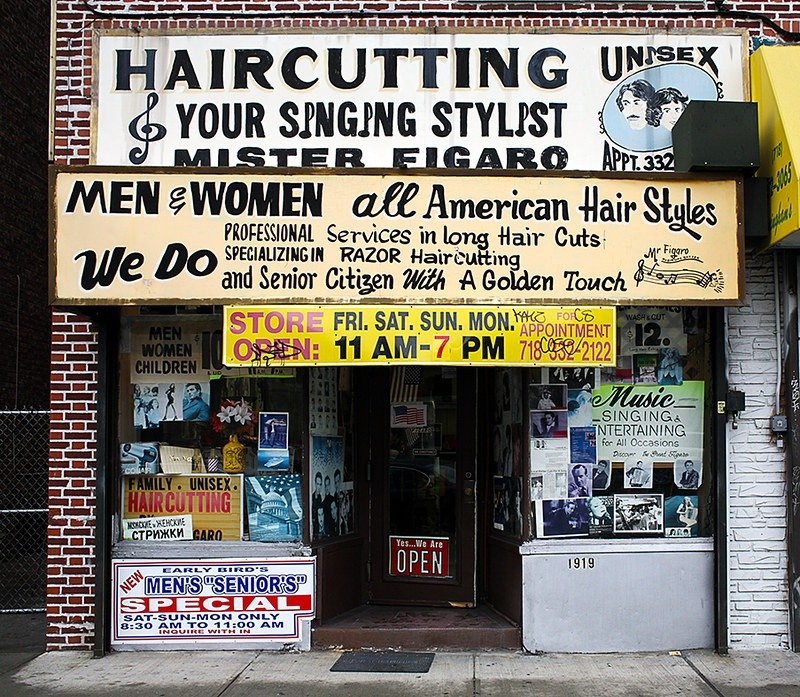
[222,435,246,473]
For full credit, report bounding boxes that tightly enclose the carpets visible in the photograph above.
[331,650,435,674]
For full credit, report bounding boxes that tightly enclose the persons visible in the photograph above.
[134,381,356,544]
[533,347,699,537]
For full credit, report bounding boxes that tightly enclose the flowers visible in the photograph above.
[213,397,254,435]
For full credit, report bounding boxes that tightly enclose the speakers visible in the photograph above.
[670,98,760,180]
